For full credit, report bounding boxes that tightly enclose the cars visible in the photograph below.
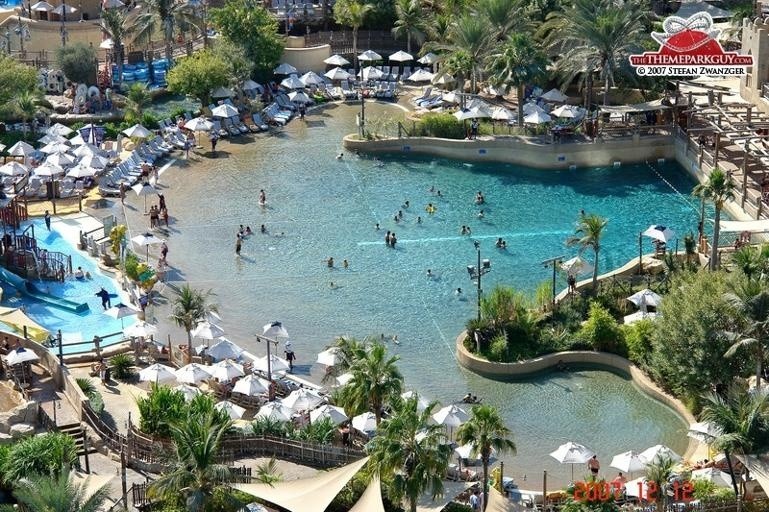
[281,1,318,16]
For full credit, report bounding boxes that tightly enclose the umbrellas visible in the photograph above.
[99,38,125,51]
[50,3,77,15]
[627,288,663,308]
[642,224,675,259]
[131,231,164,262]
[122,123,152,138]
[30,1,54,12]
[623,310,663,329]
[101,0,125,10]
[560,257,594,287]
[4,346,40,384]
[0,122,106,202]
[117,133,122,155]
[549,421,733,503]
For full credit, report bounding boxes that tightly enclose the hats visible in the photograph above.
[286,341,291,346]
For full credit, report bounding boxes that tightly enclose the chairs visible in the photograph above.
[338,65,590,141]
[158,348,287,423]
[0,61,339,200]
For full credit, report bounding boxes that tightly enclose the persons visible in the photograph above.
[567,275,575,294]
[16,290,22,297]
[119,183,125,204]
[184,138,191,156]
[1,337,9,350]
[20,303,26,314]
[45,210,51,231]
[469,491,481,512]
[234,185,507,297]
[734,239,741,249]
[479,490,489,512]
[12,339,22,349]
[336,152,343,160]
[88,357,105,377]
[211,137,217,153]
[557,360,571,373]
[74,267,84,279]
[96,286,111,310]
[376,157,381,162]
[697,222,702,244]
[1,232,48,268]
[578,210,585,215]
[15,25,95,50]
[355,152,361,157]
[139,160,169,268]
[646,109,716,148]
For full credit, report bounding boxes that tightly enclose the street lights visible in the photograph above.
[15,15,32,64]
[46,329,66,368]
[1,24,14,56]
[352,79,377,136]
